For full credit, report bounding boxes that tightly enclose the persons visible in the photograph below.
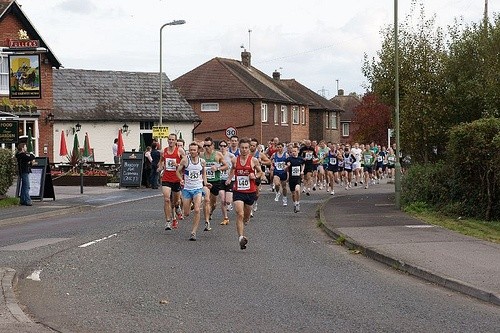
[112,138,120,164]
[15,143,35,206]
[144,133,407,249]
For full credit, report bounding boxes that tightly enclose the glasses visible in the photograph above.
[220,145,227,148]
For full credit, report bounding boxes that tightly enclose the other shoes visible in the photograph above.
[146,185,159,189]
[21,203,32,207]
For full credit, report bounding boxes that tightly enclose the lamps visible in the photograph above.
[120,123,128,134]
[45,113,54,124]
[72,121,82,134]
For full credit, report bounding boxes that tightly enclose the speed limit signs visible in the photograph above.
[224,126,237,139]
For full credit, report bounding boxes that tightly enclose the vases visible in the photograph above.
[52,176,108,186]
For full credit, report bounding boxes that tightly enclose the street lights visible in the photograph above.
[158,19,186,184]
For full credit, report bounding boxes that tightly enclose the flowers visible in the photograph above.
[52,168,108,176]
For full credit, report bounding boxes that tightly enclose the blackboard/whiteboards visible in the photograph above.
[120,152,144,187]
[16,157,54,198]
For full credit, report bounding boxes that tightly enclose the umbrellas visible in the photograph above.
[83,132,92,161]
[72,133,80,159]
[60,130,68,162]
[117,128,125,162]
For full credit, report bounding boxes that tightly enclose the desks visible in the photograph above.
[49,161,119,173]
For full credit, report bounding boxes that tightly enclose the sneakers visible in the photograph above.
[209,214,212,220]
[220,217,229,225]
[227,204,232,210]
[204,222,210,231]
[175,205,182,215]
[165,222,172,230]
[270,172,392,213]
[253,202,257,212]
[239,237,247,249]
[243,218,249,225]
[189,233,196,240]
[172,220,177,228]
[178,214,182,220]
[250,210,252,217]
